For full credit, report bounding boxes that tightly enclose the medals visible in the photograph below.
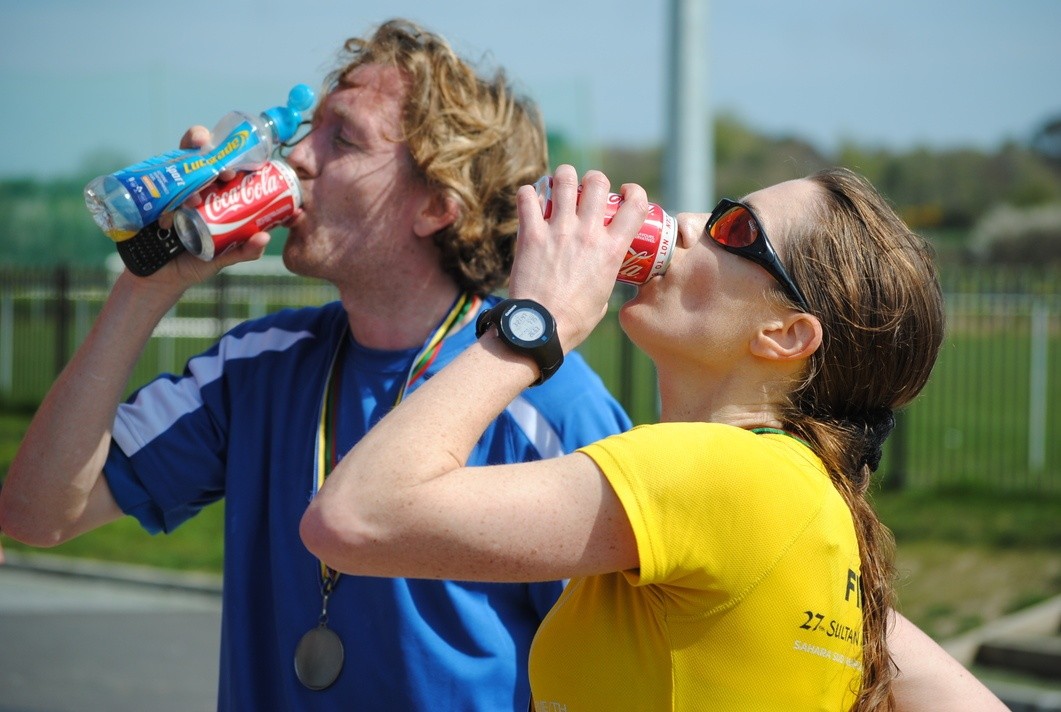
[291,622,348,694]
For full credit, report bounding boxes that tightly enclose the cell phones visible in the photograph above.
[116,220,185,276]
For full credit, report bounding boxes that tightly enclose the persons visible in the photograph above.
[0,20,1030,711]
[294,166,951,711]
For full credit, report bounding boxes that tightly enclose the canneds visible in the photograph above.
[527,177,680,285]
[172,159,300,261]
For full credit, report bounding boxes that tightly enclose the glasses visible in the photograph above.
[705,196,808,316]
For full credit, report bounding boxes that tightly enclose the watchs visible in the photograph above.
[475,297,566,388]
